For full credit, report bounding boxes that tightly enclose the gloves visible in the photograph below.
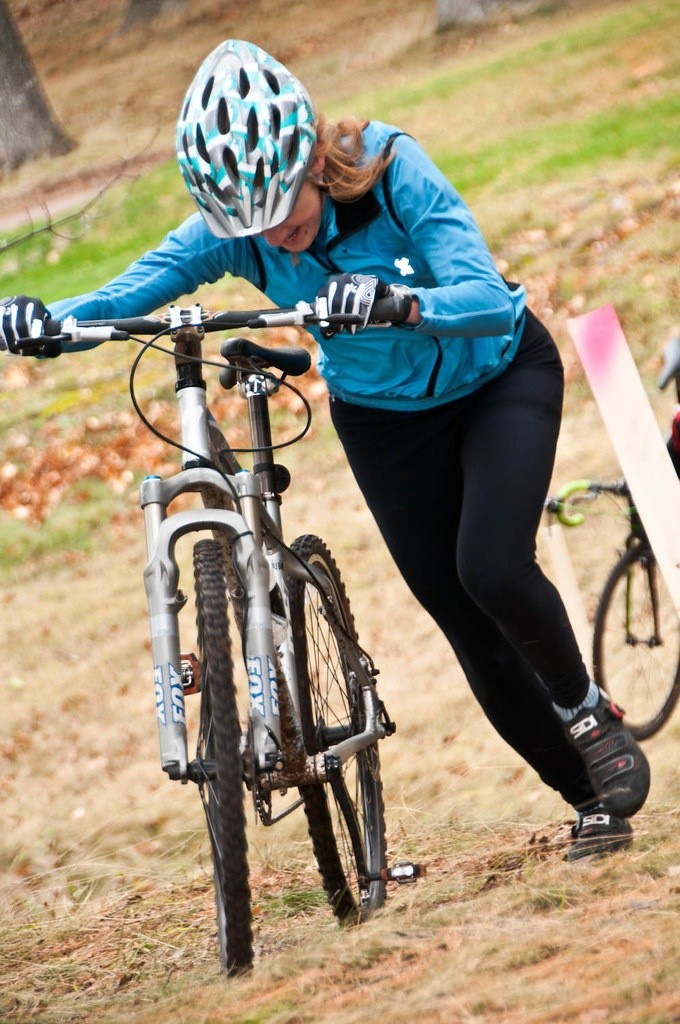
[313,271,410,336]
[0,295,54,356]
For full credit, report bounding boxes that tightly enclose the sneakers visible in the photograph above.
[567,813,632,857]
[560,691,652,817]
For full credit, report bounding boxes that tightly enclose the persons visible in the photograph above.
[0,40,651,863]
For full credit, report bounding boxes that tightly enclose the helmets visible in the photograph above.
[175,39,315,238]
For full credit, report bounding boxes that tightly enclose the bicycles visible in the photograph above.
[0,292,425,976]
[545,341,680,742]
